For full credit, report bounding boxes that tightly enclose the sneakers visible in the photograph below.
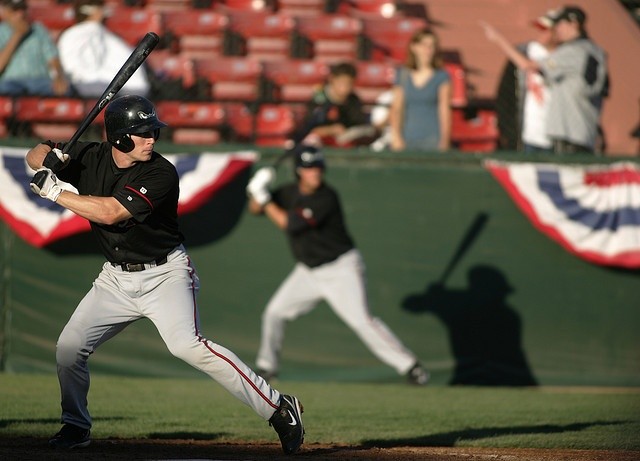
[47,423,91,449]
[254,367,277,378]
[410,365,429,383]
[269,393,305,453]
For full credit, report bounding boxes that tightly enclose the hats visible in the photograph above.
[557,9,585,20]
[534,8,560,30]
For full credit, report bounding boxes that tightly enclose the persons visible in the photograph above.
[0,0,69,98]
[288,62,376,148]
[24,96,306,455]
[245,147,430,385]
[57,1,151,99]
[480,8,609,155]
[390,28,453,151]
[496,11,560,154]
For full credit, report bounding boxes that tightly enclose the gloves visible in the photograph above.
[35,167,63,202]
[41,147,69,175]
[246,166,276,192]
[250,178,272,205]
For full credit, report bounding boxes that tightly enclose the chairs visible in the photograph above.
[0,0,502,152]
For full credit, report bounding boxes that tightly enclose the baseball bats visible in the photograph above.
[30,33,160,195]
[273,102,331,173]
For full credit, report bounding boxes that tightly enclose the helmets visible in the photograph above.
[105,94,167,152]
[295,147,324,177]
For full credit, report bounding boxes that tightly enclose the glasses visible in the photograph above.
[128,124,161,140]
[13,5,26,10]
[300,152,322,162]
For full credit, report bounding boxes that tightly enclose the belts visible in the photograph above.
[110,258,167,272]
